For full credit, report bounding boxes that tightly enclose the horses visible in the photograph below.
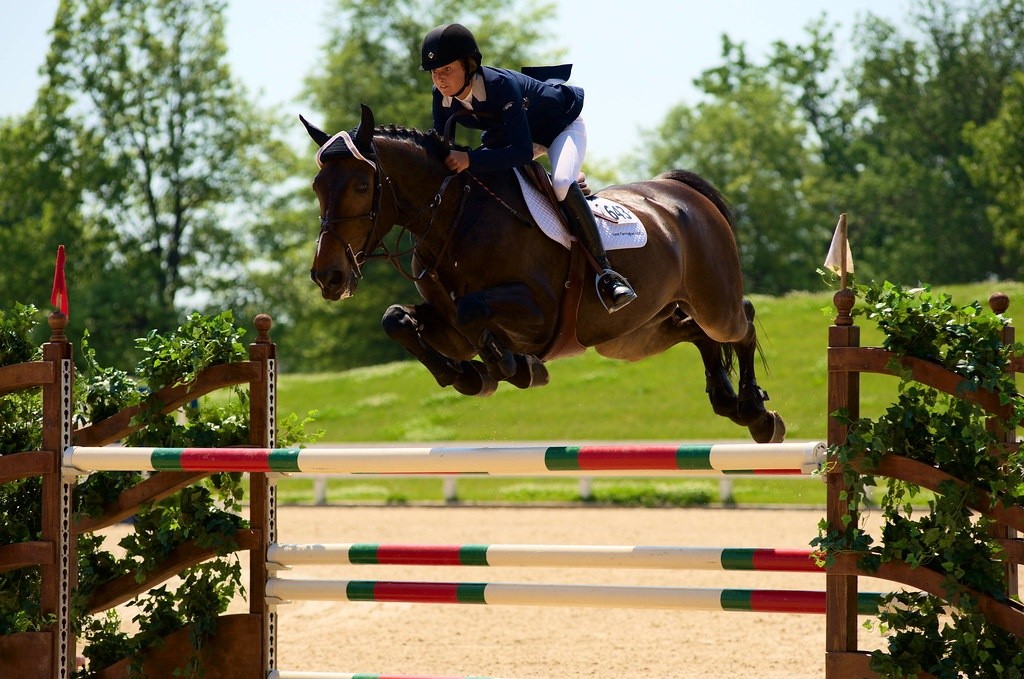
[299,104,785,443]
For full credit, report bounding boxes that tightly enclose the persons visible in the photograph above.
[419,23,638,314]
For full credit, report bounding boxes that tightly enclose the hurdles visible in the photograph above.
[1,291,1022,679]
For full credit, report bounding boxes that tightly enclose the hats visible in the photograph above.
[419,23,483,98]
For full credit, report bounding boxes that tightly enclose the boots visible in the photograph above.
[556,181,636,310]
